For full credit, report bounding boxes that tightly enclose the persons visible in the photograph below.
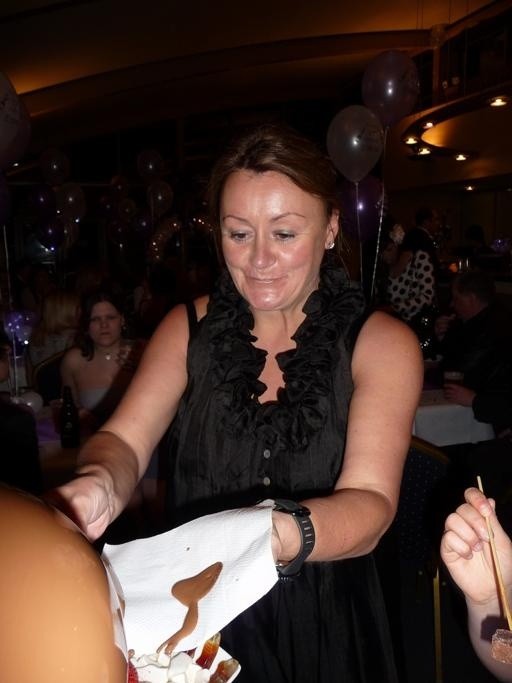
[43,116,426,681]
[1,203,512,683]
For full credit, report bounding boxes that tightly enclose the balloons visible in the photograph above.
[96,194,121,220]
[327,102,386,185]
[361,48,419,129]
[33,182,54,210]
[334,174,394,243]
[146,178,172,214]
[61,182,87,222]
[108,175,130,200]
[36,171,70,208]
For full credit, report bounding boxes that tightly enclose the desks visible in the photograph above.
[412,354,496,463]
